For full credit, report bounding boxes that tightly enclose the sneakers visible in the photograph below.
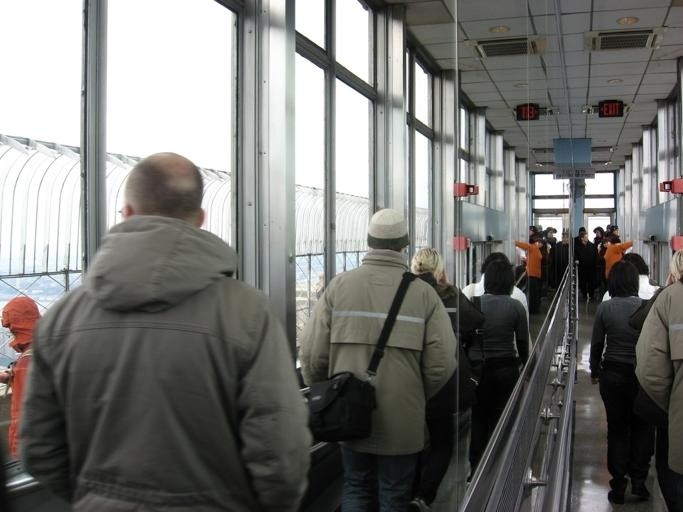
[409,496,432,511]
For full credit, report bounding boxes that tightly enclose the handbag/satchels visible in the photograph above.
[308,371,376,442]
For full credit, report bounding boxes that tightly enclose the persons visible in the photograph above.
[514,225,635,313]
[19,151,311,512]
[590,250,683,512]
[301,209,458,512]
[575,224,682,512]
[1,293,68,468]
[409,250,529,512]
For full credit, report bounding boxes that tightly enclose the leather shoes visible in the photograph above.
[608,490,624,505]
[631,483,651,500]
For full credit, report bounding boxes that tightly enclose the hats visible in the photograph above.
[367,209,409,250]
[610,226,618,233]
